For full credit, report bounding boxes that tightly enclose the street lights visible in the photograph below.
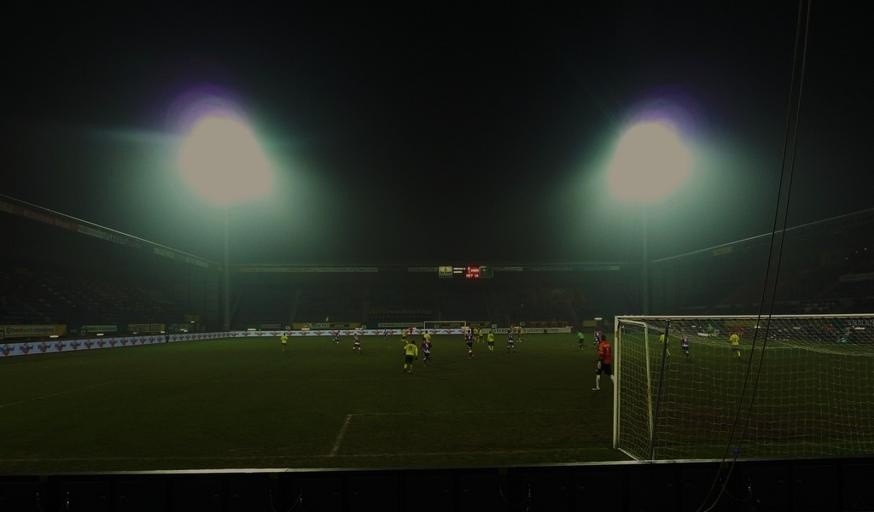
[601,116,699,317]
[181,113,268,334]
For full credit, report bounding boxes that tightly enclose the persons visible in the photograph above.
[352,333,360,351]
[592,329,602,352]
[731,332,741,360]
[576,330,585,351]
[400,330,433,374]
[464,327,495,360]
[383,328,393,337]
[590,333,614,391]
[506,330,514,349]
[658,332,671,358]
[680,333,690,356]
[280,333,288,351]
